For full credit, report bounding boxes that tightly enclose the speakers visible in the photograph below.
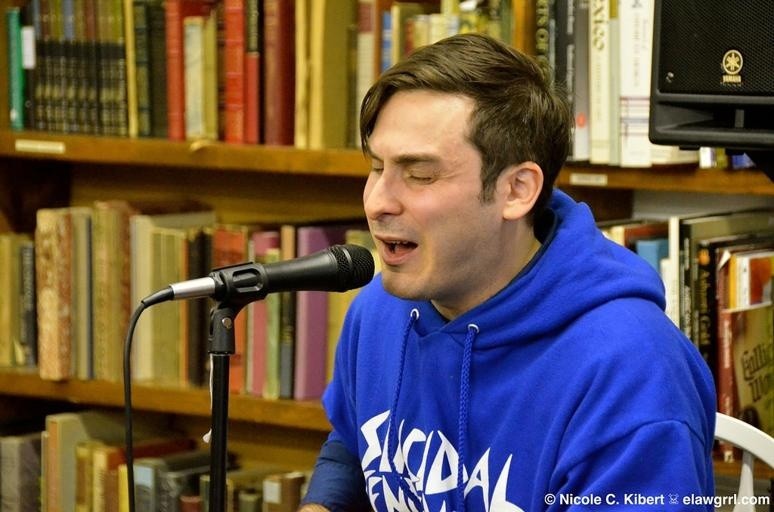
[648,0,774,151]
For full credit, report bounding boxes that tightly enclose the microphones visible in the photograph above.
[172,244,375,301]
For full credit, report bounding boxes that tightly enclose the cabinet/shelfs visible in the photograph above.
[0,0,773,480]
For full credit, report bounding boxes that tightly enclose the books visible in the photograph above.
[0,1,773,512]
